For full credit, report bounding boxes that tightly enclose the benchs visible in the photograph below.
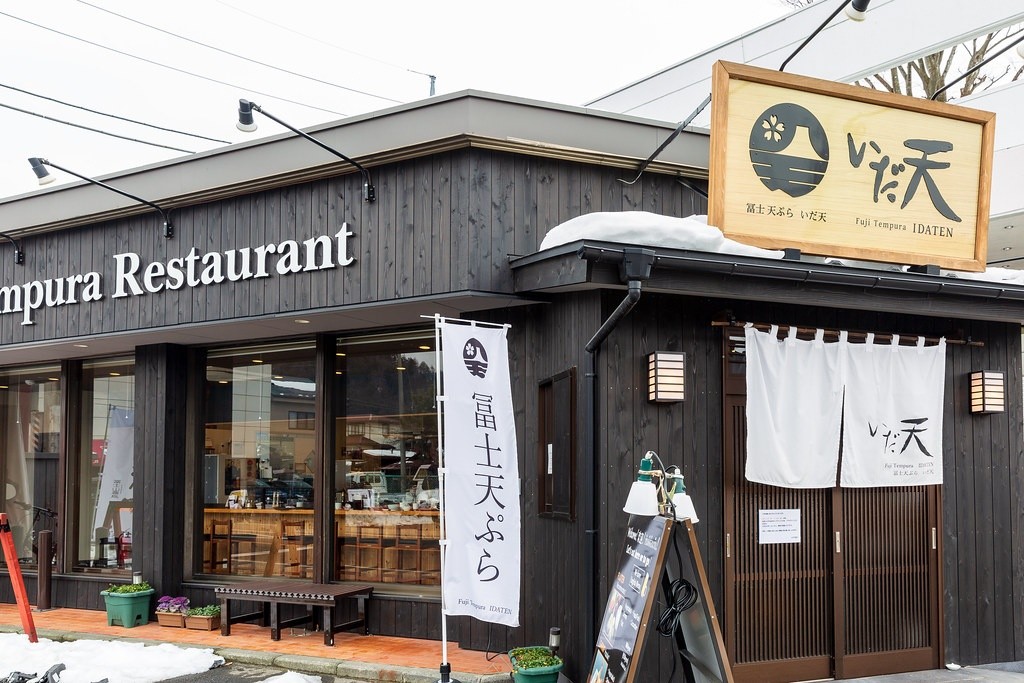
[215,579,374,646]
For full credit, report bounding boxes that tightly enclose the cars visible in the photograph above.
[266,480,314,503]
[225,476,288,505]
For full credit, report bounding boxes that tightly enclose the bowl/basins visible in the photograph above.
[388,505,399,511]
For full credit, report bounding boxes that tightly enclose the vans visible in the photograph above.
[345,470,388,494]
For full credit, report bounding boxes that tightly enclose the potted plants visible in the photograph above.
[183,605,220,631]
[100,581,154,628]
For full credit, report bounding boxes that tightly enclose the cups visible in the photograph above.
[335,503,342,510]
[297,502,303,507]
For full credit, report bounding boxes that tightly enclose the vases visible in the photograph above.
[155,610,186,628]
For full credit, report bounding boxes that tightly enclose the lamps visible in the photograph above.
[644,350,687,403]
[623,457,659,516]
[549,627,561,658]
[968,370,1006,414]
[668,469,700,525]
[779,0,869,72]
[236,99,375,202]
[28,157,173,238]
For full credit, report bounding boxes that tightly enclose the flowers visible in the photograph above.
[157,596,190,614]
